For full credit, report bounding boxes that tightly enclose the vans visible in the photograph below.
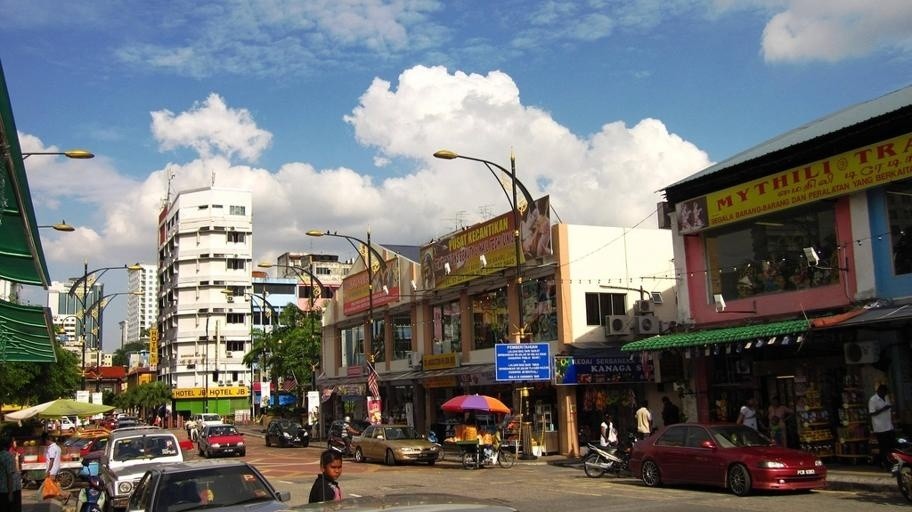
[190,412,224,434]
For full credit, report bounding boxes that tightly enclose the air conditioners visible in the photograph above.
[606,314,630,336]
[634,316,659,334]
[635,300,654,312]
[227,295,234,301]
[218,379,246,386]
[227,351,232,356]
[406,352,421,366]
[844,338,879,365]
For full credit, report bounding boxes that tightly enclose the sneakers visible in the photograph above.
[62,492,71,505]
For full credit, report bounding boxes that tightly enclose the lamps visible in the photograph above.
[444,261,489,277]
[409,279,434,292]
[803,247,848,271]
[712,294,758,314]
[383,285,428,297]
[478,254,526,268]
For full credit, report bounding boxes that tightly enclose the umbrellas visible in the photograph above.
[4,397,116,443]
[440,393,510,415]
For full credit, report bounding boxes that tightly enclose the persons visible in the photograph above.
[660,395,682,428]
[599,414,619,448]
[680,204,692,230]
[0,420,62,512]
[692,202,703,226]
[737,395,795,448]
[309,449,343,503]
[868,383,897,469]
[633,399,653,441]
[153,414,204,441]
[341,416,360,458]
[466,410,482,431]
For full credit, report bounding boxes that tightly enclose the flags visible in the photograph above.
[366,362,380,400]
[283,373,297,392]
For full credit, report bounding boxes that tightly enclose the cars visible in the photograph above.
[126,457,292,512]
[266,419,310,448]
[197,424,246,458]
[286,491,521,512]
[629,423,828,497]
[19,413,151,491]
[349,424,439,466]
[327,419,373,456]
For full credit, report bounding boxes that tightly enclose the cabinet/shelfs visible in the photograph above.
[502,414,523,453]
[797,389,833,458]
[534,403,558,455]
[835,386,870,463]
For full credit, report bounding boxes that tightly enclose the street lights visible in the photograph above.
[10,221,75,304]
[220,288,280,416]
[87,289,146,426]
[433,146,536,461]
[256,264,328,434]
[304,229,387,424]
[59,261,145,427]
[21,149,95,161]
[121,364,139,386]
[206,312,213,413]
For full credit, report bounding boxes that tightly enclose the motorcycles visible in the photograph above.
[189,425,198,442]
[887,437,911,505]
[327,430,365,459]
[74,458,109,512]
[412,425,445,462]
[583,426,657,478]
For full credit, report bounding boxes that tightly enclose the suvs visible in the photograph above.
[97,426,183,512]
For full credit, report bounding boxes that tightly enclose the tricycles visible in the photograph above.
[456,422,515,470]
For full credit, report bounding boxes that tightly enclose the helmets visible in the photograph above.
[344,416,351,423]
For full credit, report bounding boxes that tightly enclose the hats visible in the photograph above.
[86,475,106,491]
